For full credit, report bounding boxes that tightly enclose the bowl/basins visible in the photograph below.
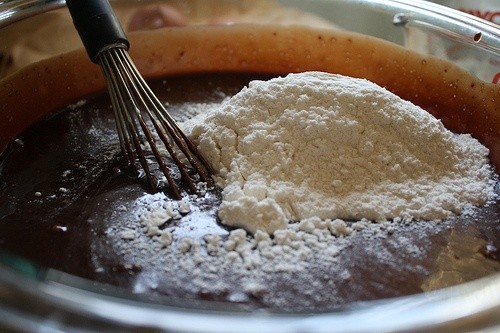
[0,0,500,333]
